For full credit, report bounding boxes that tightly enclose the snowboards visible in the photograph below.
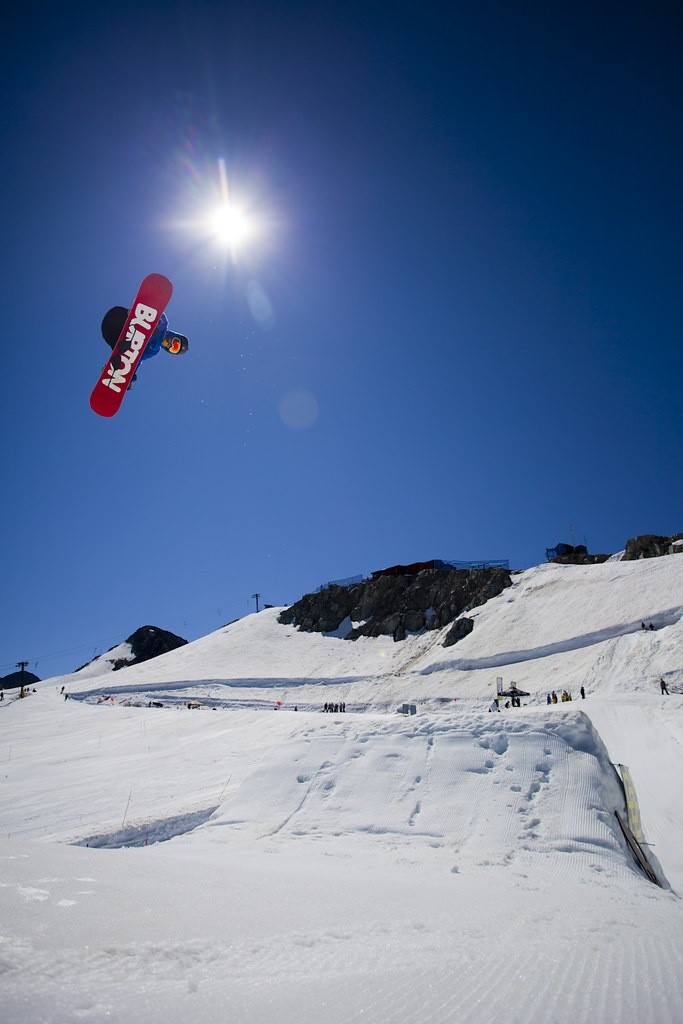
[89,274,173,418]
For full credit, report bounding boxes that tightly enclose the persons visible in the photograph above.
[101,305,190,382]
[0,622,670,716]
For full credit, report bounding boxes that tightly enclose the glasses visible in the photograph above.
[169,336,180,353]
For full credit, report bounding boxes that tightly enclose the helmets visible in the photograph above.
[169,333,189,356]
